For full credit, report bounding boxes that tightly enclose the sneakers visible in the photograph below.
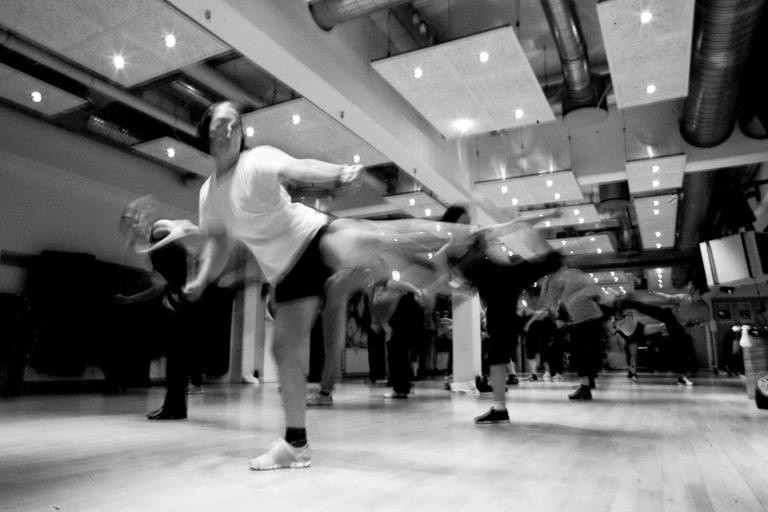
[677,375,693,387]
[507,369,564,385]
[147,403,188,420]
[568,384,592,400]
[475,407,510,425]
[249,437,314,471]
[382,389,408,400]
[305,392,334,406]
